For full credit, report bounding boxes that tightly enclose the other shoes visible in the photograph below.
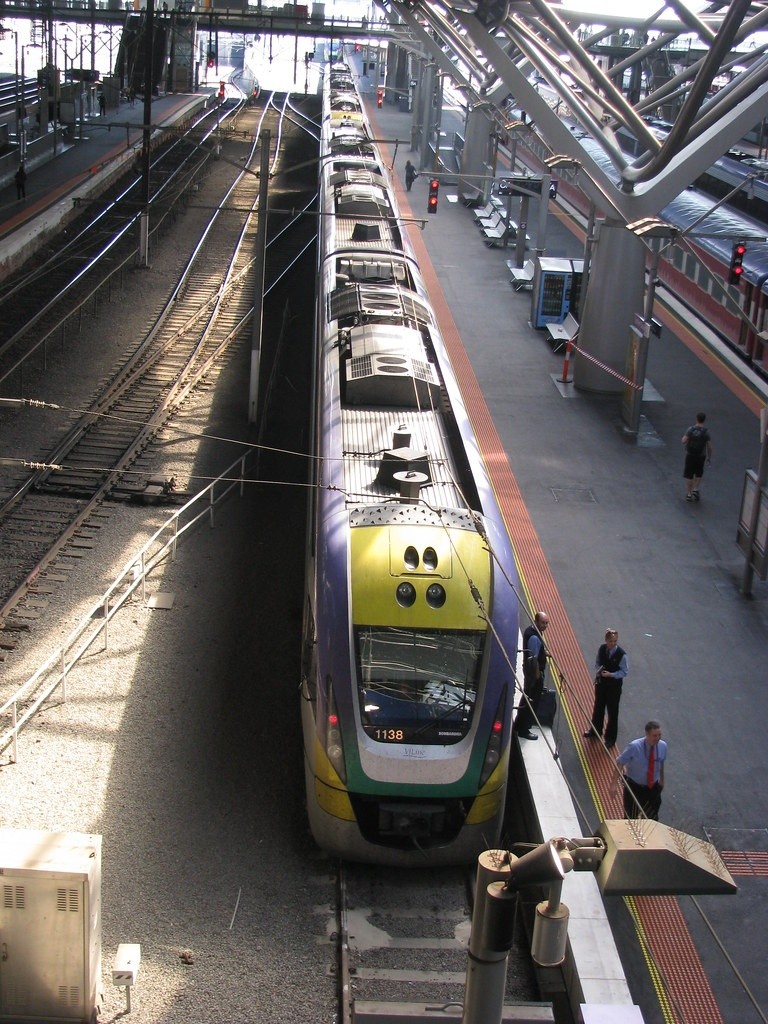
[686,494,691,501]
[692,490,699,501]
[518,730,538,740]
[605,742,615,749]
[584,730,601,738]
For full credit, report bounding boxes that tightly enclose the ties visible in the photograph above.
[647,746,655,789]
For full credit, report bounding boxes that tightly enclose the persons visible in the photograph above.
[609,721,667,822]
[405,160,416,192]
[361,15,367,28]
[583,628,627,749]
[125,0,129,10]
[514,611,553,741]
[129,88,136,107]
[162,2,169,19]
[97,93,107,116]
[15,167,27,202]
[574,25,664,51]
[681,412,712,503]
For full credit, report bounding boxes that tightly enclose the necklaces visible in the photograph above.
[644,739,659,762]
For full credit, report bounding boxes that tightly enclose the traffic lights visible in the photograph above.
[254,91,256,96]
[378,90,382,108]
[727,243,747,285]
[220,81,224,96]
[254,84,257,88]
[355,43,359,54]
[207,52,214,68]
[427,179,440,213]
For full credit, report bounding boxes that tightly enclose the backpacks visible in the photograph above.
[687,428,708,456]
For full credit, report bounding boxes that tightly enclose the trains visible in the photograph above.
[523,65,768,219]
[440,58,768,379]
[300,60,520,866]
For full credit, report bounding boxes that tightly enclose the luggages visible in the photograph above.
[534,655,557,728]
